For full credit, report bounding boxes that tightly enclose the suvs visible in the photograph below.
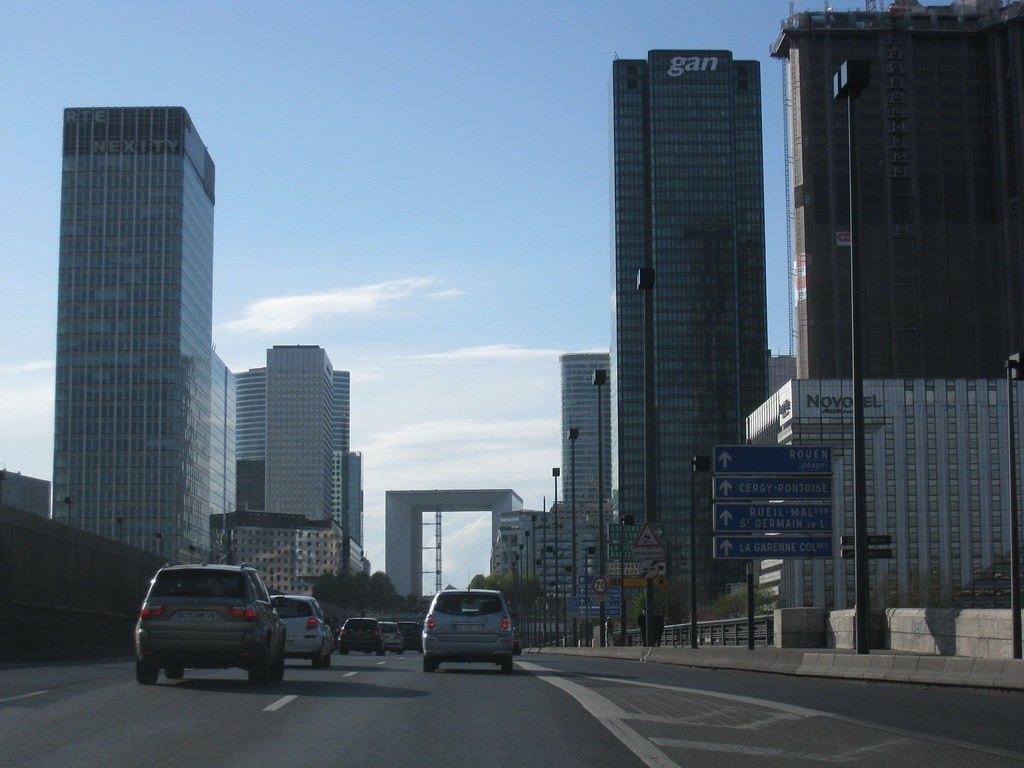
[421,588,516,674]
[338,617,387,657]
[137,560,290,691]
[377,620,405,655]
[395,620,424,654]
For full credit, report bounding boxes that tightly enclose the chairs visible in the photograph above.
[442,600,462,612]
[483,601,498,613]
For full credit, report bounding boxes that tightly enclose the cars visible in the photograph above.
[266,594,337,667]
[512,630,522,657]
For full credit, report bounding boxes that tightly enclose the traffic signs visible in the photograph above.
[712,444,836,476]
[711,474,833,500]
[712,535,833,559]
[712,501,834,533]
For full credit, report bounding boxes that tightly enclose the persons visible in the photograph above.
[637,608,665,647]
[605,617,614,647]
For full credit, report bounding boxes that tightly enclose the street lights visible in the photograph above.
[832,57,873,656]
[552,467,560,648]
[567,428,578,647]
[591,369,607,647]
[531,514,537,647]
[519,543,523,648]
[525,530,530,648]
[636,266,657,649]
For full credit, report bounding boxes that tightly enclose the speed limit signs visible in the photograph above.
[593,575,608,593]
